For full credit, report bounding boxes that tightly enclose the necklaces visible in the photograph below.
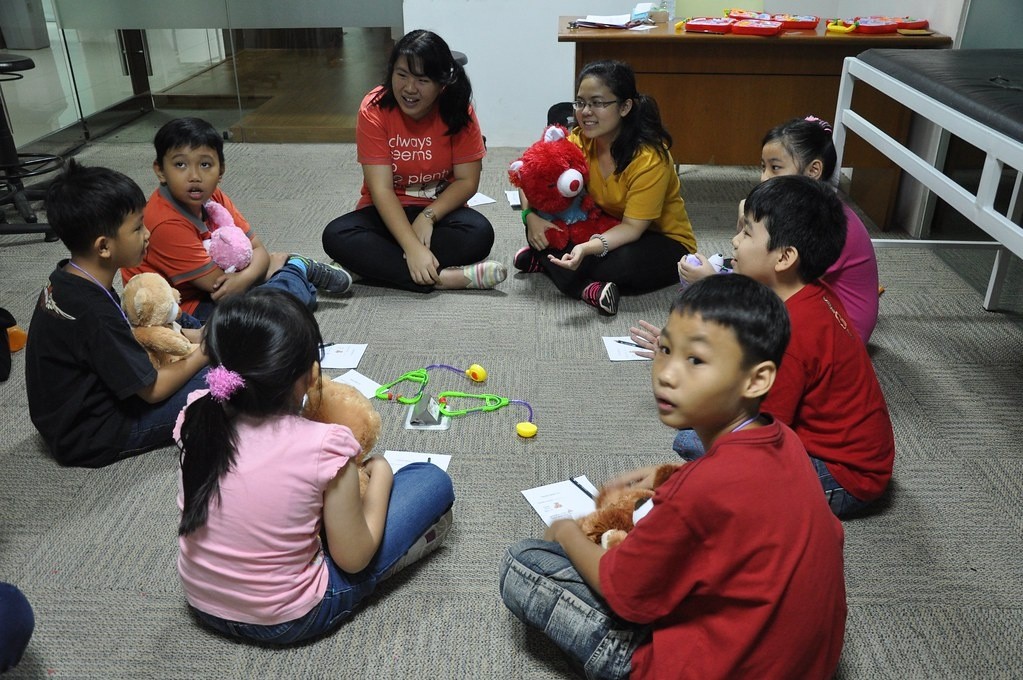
[730,412,762,432]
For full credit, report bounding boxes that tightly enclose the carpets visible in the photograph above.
[105,94,273,110]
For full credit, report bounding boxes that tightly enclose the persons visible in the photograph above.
[322,29,507,293]
[500,273,847,680]
[678,114,880,350]
[678,175,895,521]
[121,116,351,316]
[513,59,698,317]
[25,165,217,469]
[172,286,455,651]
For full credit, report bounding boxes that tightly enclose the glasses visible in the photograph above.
[572,100,616,110]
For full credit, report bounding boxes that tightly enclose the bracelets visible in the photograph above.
[590,233,608,257]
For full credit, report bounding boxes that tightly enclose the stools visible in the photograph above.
[0,52,67,242]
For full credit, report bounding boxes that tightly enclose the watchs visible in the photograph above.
[423,208,437,224]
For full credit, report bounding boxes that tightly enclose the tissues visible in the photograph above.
[630,2,669,24]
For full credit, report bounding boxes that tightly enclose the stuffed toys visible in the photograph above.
[574,463,682,550]
[203,201,253,274]
[121,273,201,369]
[508,123,621,249]
[299,372,382,502]
[679,254,733,289]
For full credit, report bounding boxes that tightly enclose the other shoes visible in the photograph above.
[377,509,452,583]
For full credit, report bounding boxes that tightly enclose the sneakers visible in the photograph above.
[447,260,506,290]
[284,252,352,293]
[583,282,618,314]
[513,247,544,272]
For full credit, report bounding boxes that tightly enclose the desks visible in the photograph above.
[558,15,952,234]
[826,47,1023,312]
